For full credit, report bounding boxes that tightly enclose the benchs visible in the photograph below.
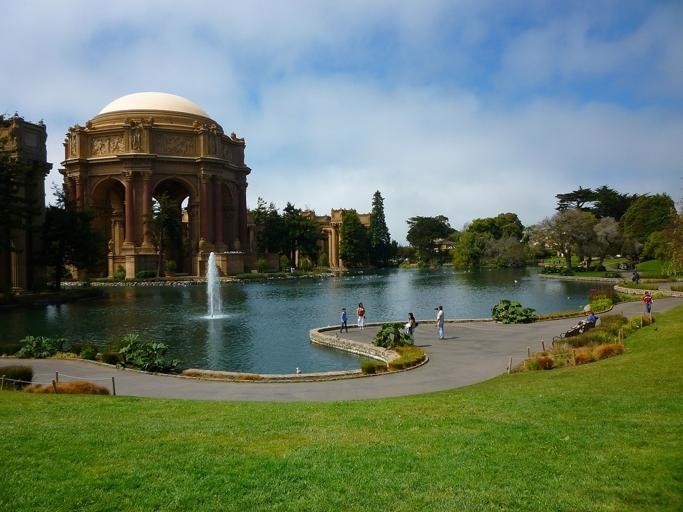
[552,321,595,343]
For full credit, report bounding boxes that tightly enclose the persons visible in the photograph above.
[340,307,349,334]
[433,305,444,340]
[404,313,416,345]
[631,271,639,284]
[356,302,366,330]
[641,291,653,314]
[572,312,596,329]
[111,137,120,152]
[92,138,104,155]
